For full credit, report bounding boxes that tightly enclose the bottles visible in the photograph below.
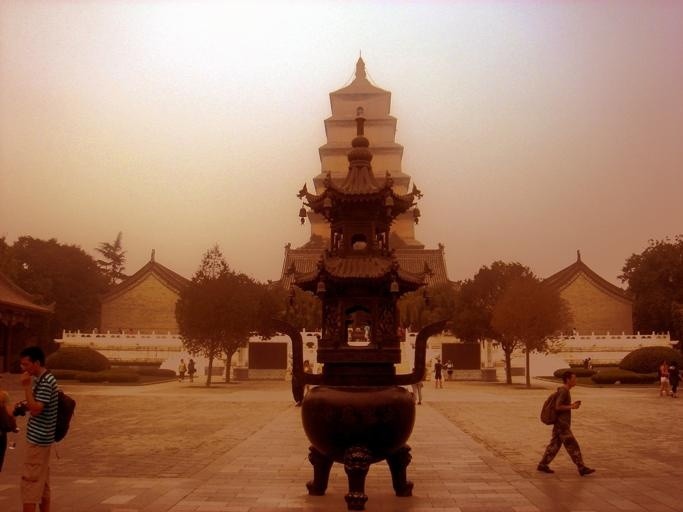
[8,424,20,450]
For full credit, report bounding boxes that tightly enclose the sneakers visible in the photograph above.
[538,464,555,474]
[578,466,595,476]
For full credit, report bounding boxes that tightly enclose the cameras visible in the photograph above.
[12,403,25,416]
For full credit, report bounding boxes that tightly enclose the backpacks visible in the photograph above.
[55,390,76,443]
[541,387,561,425]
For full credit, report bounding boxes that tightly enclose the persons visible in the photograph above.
[188,359,194,381]
[537,372,595,475]
[0,390,19,472]
[303,360,312,390]
[668,362,679,398]
[16,346,59,512]
[412,368,423,404]
[443,359,454,380]
[434,359,443,388]
[660,361,668,397]
[179,358,186,380]
